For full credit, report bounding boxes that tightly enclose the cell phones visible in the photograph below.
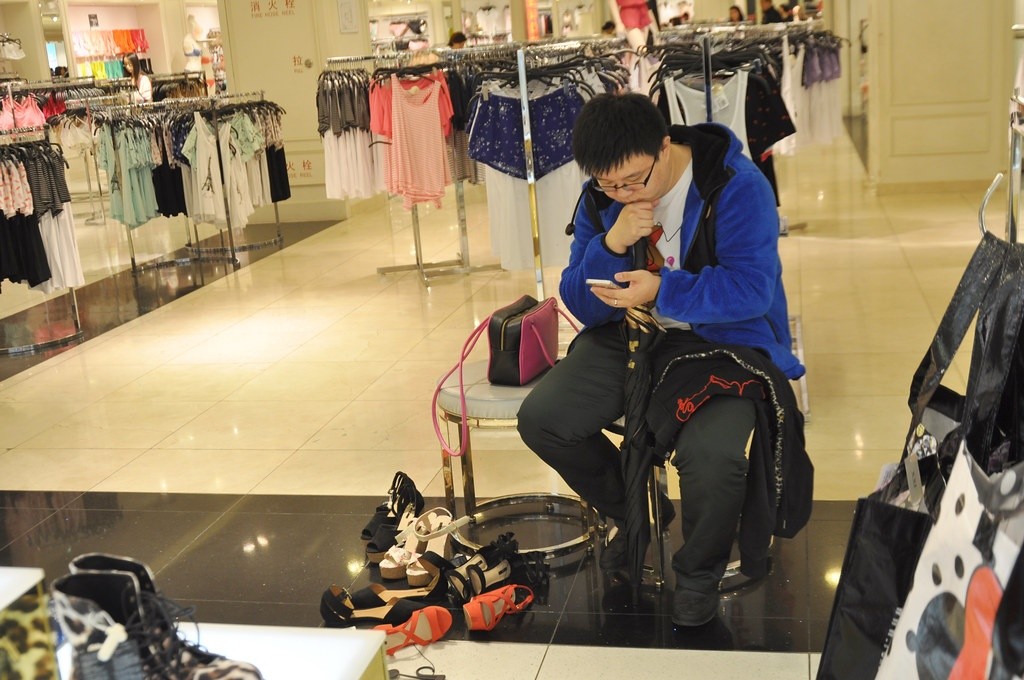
[585,279,623,291]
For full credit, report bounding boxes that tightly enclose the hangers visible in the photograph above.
[315,17,852,142]
[0,32,287,173]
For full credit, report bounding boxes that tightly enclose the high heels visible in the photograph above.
[318,470,553,678]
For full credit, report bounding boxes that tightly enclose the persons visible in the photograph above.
[514,92,809,627]
[55,66,65,76]
[446,0,795,50]
[181,13,216,98]
[121,52,153,104]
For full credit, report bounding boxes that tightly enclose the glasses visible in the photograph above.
[592,152,658,193]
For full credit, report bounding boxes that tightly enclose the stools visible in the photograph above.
[606,417,778,595]
[433,354,613,566]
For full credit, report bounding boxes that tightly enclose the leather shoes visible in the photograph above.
[670,587,721,626]
[600,492,676,573]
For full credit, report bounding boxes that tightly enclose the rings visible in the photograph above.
[614,299,618,306]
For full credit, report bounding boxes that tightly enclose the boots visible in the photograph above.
[69,550,262,680]
[49,570,181,680]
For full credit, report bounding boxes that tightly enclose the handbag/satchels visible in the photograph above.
[488,294,581,387]
[815,231,1024,680]
